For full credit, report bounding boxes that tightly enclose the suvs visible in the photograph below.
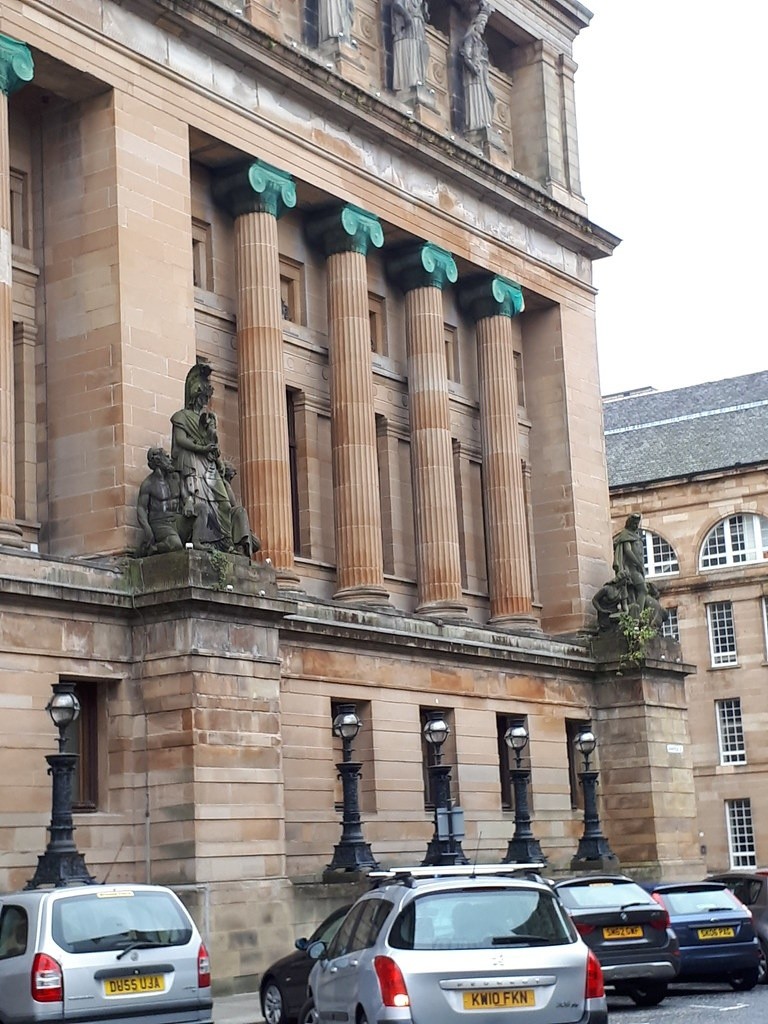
[544,873,681,1006]
[701,867,768,983]
[298,862,608,1024]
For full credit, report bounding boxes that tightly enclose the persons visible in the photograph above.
[137,364,260,554]
[457,12,496,129]
[592,514,670,632]
[388,0,435,90]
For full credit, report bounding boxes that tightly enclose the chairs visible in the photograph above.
[416,917,435,941]
[474,903,519,935]
[14,922,28,946]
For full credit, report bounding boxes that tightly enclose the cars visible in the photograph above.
[638,882,761,990]
[0,884,215,1024]
[258,903,353,1024]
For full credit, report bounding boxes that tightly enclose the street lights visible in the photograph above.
[422,711,470,867]
[500,718,551,865]
[23,683,97,885]
[574,726,622,864]
[326,702,381,871]
